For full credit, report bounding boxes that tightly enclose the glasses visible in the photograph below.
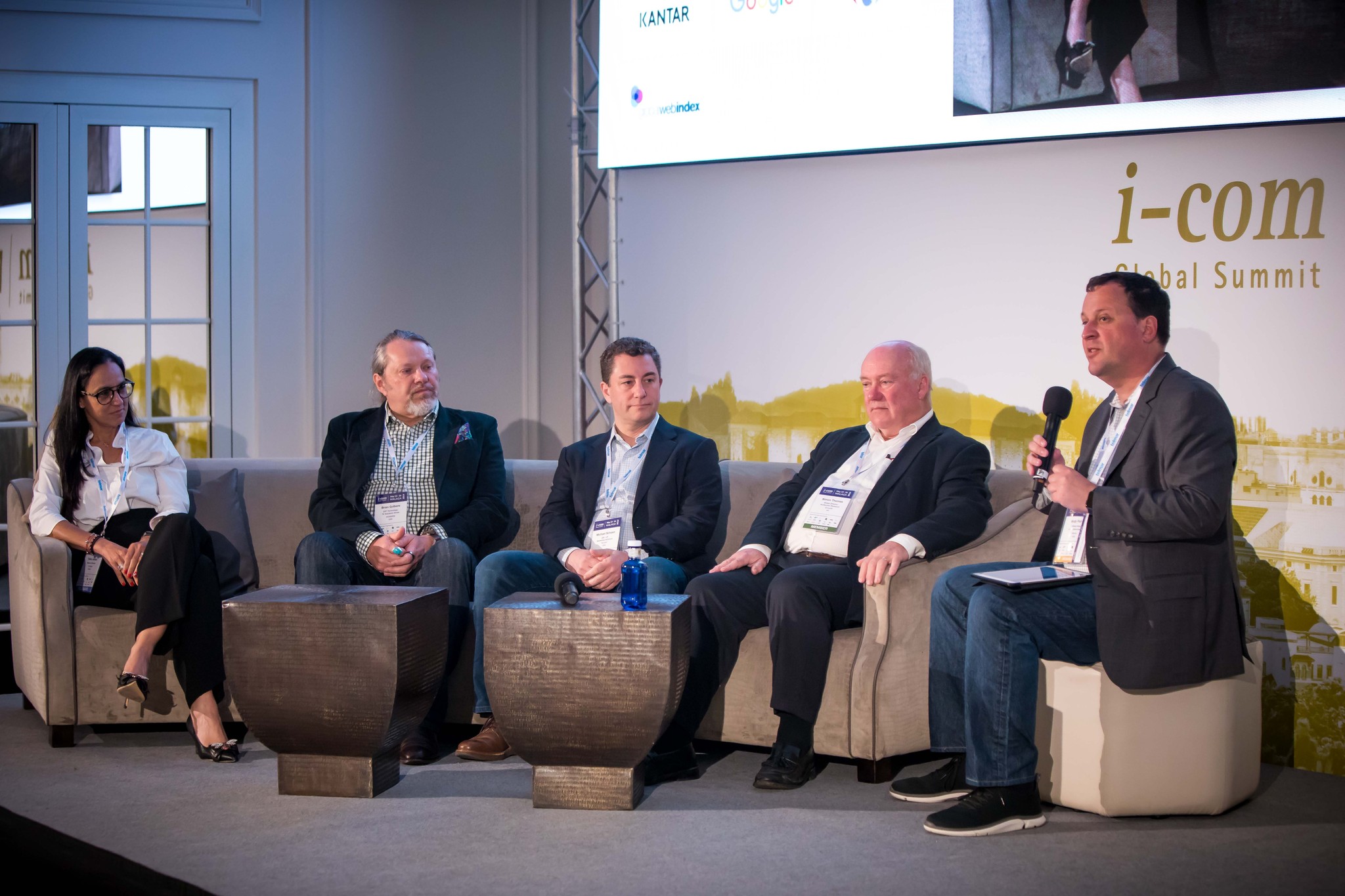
[81,378,136,405]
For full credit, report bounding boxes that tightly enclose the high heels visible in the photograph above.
[186,714,240,762]
[116,670,149,707]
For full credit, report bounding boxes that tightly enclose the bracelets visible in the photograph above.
[85,533,104,556]
[421,529,441,544]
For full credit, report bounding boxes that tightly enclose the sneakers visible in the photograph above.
[888,754,975,802]
[922,773,1045,836]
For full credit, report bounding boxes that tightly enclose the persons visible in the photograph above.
[1052,0,1148,104]
[294,327,509,765]
[644,338,993,789]
[889,271,1257,837]
[29,346,241,762]
[456,336,721,761]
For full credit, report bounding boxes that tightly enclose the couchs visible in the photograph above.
[7,456,1047,784]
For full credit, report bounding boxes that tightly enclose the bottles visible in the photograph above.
[620,540,648,612]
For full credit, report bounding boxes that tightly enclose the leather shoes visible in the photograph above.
[455,716,517,763]
[752,739,818,789]
[642,740,701,786]
[400,732,430,765]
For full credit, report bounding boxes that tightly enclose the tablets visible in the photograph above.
[970,565,1093,592]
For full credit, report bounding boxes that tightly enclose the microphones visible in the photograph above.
[1033,386,1073,495]
[555,571,583,606]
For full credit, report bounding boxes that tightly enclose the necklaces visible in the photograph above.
[94,430,117,448]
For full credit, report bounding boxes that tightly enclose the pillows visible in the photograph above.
[187,468,255,593]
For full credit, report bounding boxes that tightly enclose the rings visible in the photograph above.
[405,551,416,559]
[392,546,403,556]
[118,563,123,571]
[139,552,144,558]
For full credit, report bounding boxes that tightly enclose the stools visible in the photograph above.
[1036,640,1263,817]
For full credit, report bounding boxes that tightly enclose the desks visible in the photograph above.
[484,592,692,811]
[223,585,448,798]
